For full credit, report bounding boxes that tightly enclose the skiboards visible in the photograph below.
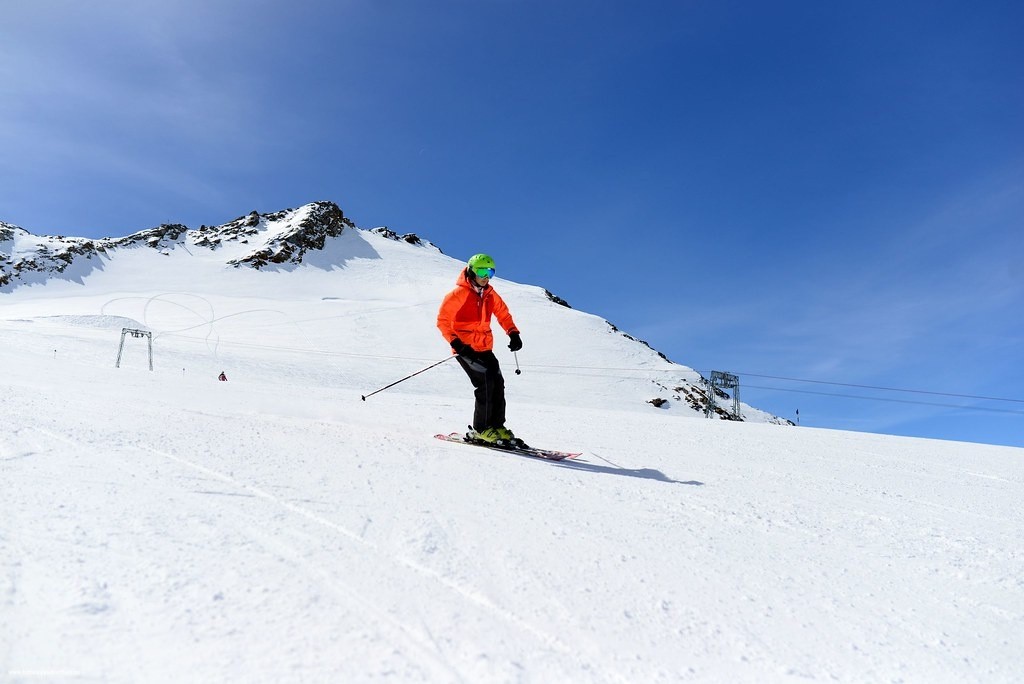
[434,432,584,461]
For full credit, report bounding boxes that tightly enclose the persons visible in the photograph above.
[437,254,523,442]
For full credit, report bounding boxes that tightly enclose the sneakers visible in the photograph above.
[472,427,501,443]
[496,425,514,442]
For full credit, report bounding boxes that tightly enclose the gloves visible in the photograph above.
[451,337,474,356]
[508,332,522,351]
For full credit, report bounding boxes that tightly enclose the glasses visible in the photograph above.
[474,267,495,279]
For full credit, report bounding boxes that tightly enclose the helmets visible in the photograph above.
[468,254,495,277]
